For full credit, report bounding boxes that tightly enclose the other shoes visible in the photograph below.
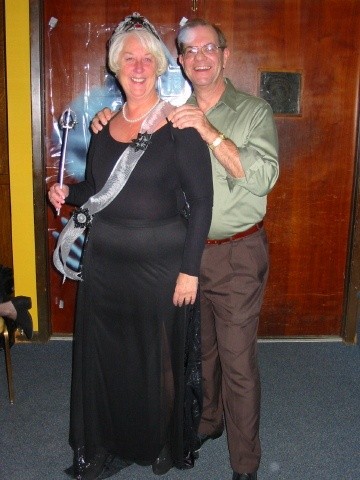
[233,470,257,480]
[152,445,171,475]
[196,430,222,450]
[76,447,112,480]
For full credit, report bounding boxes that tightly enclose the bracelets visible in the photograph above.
[210,132,225,152]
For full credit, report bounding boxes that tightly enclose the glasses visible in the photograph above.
[182,43,224,56]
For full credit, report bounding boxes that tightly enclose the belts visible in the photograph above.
[206,220,264,246]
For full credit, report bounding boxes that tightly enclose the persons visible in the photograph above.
[89,19,280,480]
[49,12,215,480]
[0,296,34,347]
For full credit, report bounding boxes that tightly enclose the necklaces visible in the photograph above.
[122,97,162,123]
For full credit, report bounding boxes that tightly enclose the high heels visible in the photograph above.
[2,316,16,348]
[11,296,33,340]
